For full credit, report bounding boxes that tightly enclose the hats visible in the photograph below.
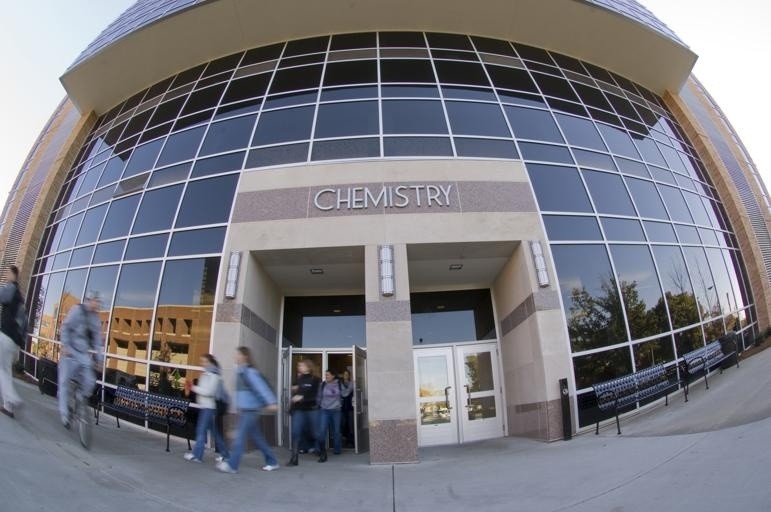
[84,290,102,301]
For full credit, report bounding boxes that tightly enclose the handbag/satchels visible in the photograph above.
[216,380,230,416]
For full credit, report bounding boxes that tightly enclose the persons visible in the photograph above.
[0,264,31,416]
[54,289,109,429]
[214,347,282,475]
[315,368,351,455]
[286,358,328,465]
[179,353,231,463]
[339,370,360,441]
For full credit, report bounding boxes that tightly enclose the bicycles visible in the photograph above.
[61,344,98,448]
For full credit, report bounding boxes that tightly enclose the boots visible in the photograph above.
[285,439,298,467]
[316,439,328,463]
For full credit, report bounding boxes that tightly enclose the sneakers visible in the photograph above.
[262,461,280,471]
[184,452,202,463]
[215,456,238,473]
